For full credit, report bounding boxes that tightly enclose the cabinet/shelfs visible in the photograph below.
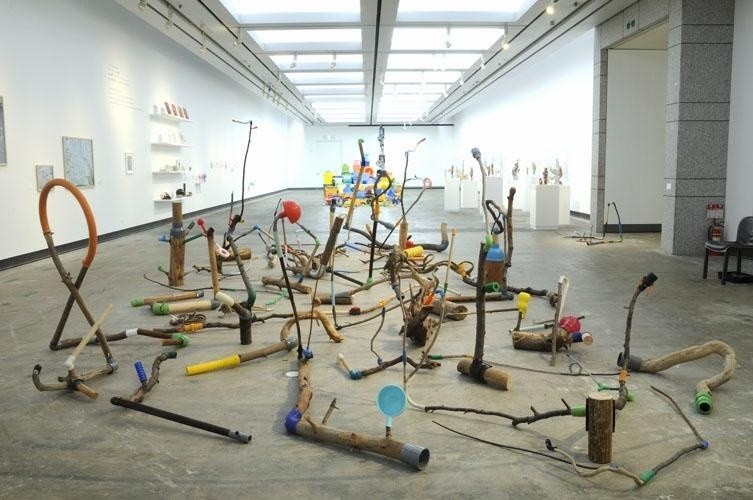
[150,113,193,203]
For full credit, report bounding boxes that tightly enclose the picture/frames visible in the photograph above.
[62,136,95,189]
[125,152,134,176]
[36,165,55,193]
[0,96,8,166]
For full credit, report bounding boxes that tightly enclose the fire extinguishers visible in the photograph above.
[708,218,725,256]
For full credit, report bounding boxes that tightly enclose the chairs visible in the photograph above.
[702,216,753,285]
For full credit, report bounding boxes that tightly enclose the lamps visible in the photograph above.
[136,0,559,129]
